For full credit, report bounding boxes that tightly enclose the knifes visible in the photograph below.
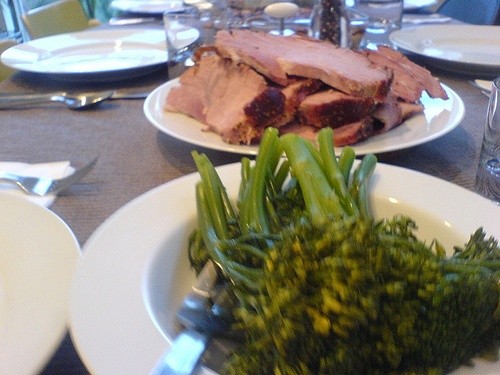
[0,89,154,101]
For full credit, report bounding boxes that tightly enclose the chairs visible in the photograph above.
[25,1,101,38]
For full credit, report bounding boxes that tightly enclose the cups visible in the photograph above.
[163,9,212,77]
[474,77,500,202]
[343,0,402,48]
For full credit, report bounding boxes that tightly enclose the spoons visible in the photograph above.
[0,89,115,111]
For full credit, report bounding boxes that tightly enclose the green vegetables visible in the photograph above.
[186,126,500,375]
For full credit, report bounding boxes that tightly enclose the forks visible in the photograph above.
[152,259,244,374]
[1,152,100,198]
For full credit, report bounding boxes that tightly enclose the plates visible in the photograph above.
[110,1,191,15]
[390,24,500,80]
[144,77,466,156]
[68,157,500,375]
[0,190,82,374]
[1,25,201,82]
